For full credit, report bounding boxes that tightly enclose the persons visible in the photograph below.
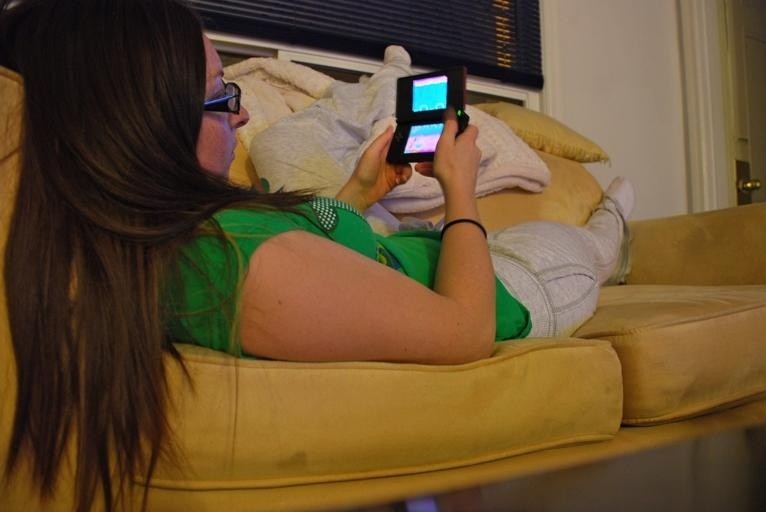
[0,0,634,511]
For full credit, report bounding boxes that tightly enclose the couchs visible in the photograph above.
[0,66,766,512]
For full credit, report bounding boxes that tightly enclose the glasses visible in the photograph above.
[204,77,241,113]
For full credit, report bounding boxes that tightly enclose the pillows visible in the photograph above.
[471,100,610,164]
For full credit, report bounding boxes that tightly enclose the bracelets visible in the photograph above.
[438,217,487,243]
